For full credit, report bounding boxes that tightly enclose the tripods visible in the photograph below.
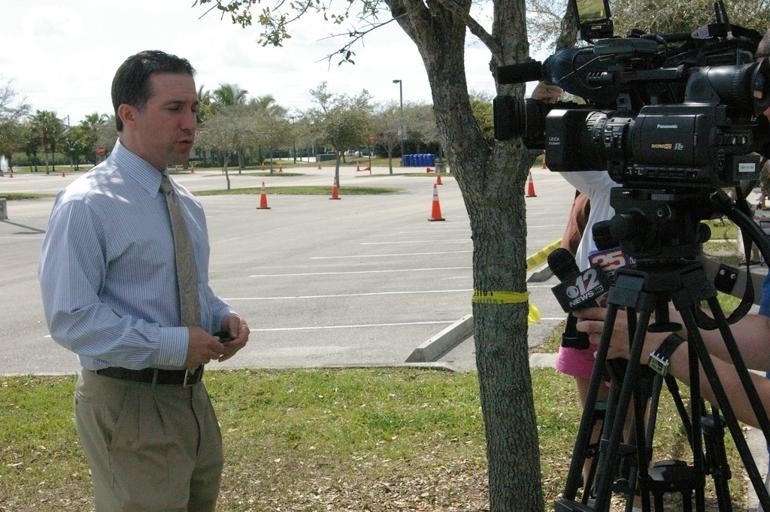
[556,265,770,512]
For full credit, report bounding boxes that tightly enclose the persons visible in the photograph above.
[570,255,770,445]
[33,44,253,509]
[526,76,657,511]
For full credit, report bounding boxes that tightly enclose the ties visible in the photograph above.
[158,174,202,375]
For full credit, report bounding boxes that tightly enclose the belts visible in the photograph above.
[90,364,204,387]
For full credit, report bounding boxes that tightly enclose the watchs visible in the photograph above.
[645,332,687,379]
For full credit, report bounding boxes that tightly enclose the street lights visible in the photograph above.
[392,80,404,156]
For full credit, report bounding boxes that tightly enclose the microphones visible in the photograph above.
[589,220,633,271]
[548,247,635,395]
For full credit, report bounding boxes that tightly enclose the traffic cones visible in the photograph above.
[329,176,341,200]
[356,159,370,171]
[525,172,538,197]
[542,159,547,169]
[426,167,446,221]
[256,181,271,209]
